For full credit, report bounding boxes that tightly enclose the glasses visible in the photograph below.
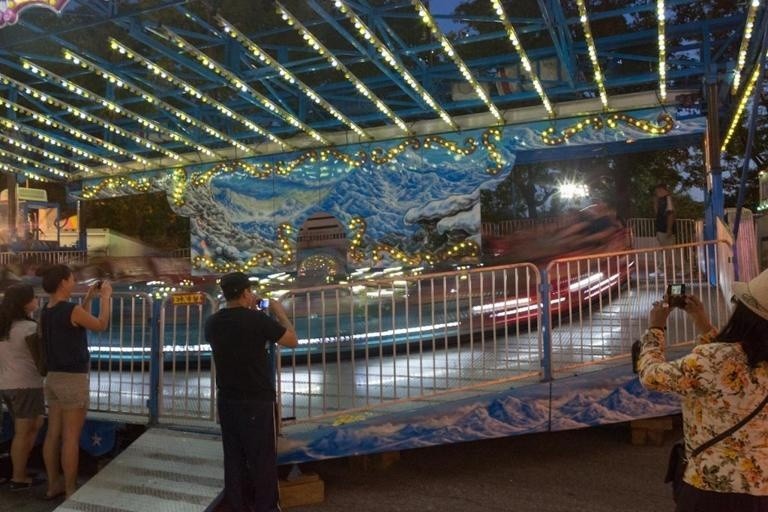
[730,294,739,304]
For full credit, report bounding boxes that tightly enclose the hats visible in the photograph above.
[220,273,259,294]
[730,269,768,321]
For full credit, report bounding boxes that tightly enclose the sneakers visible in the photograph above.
[648,271,664,278]
[676,270,689,277]
[8,477,46,492]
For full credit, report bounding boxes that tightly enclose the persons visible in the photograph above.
[648,183,697,277]
[632,266,768,509]
[200,272,300,510]
[0,284,46,490]
[34,262,114,497]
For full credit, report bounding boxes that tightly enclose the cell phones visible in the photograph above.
[258,299,270,308]
[668,284,687,306]
[95,281,103,289]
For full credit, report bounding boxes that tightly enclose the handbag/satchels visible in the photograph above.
[663,438,692,505]
